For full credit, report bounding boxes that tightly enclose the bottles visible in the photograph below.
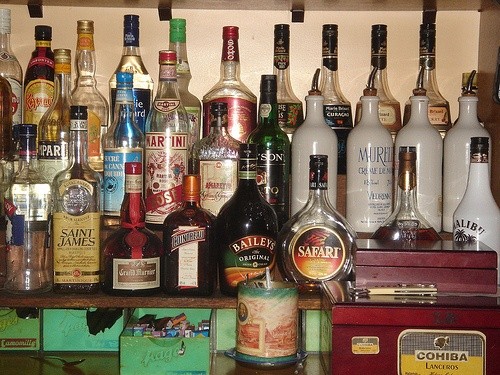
[162,174,219,296]
[394,96,443,233]
[4,123,52,296]
[202,26,257,143]
[245,74,290,231]
[217,143,279,297]
[346,96,394,233]
[290,95,338,219]
[452,137,500,285]
[318,24,353,175]
[355,24,402,147]
[51,106,102,295]
[370,145,443,239]
[443,92,491,233]
[0,8,202,230]
[259,24,304,143]
[187,102,244,217]
[276,155,359,293]
[403,23,451,141]
[100,162,163,297]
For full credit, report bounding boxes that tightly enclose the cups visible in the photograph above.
[236,280,299,363]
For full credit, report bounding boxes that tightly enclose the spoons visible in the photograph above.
[30,355,86,365]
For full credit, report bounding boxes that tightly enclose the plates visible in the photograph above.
[224,348,309,366]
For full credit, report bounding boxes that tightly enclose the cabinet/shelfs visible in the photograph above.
[0,224,457,375]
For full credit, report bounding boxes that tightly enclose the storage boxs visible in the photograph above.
[118,308,218,375]
[353,240,496,294]
[320,282,500,375]
